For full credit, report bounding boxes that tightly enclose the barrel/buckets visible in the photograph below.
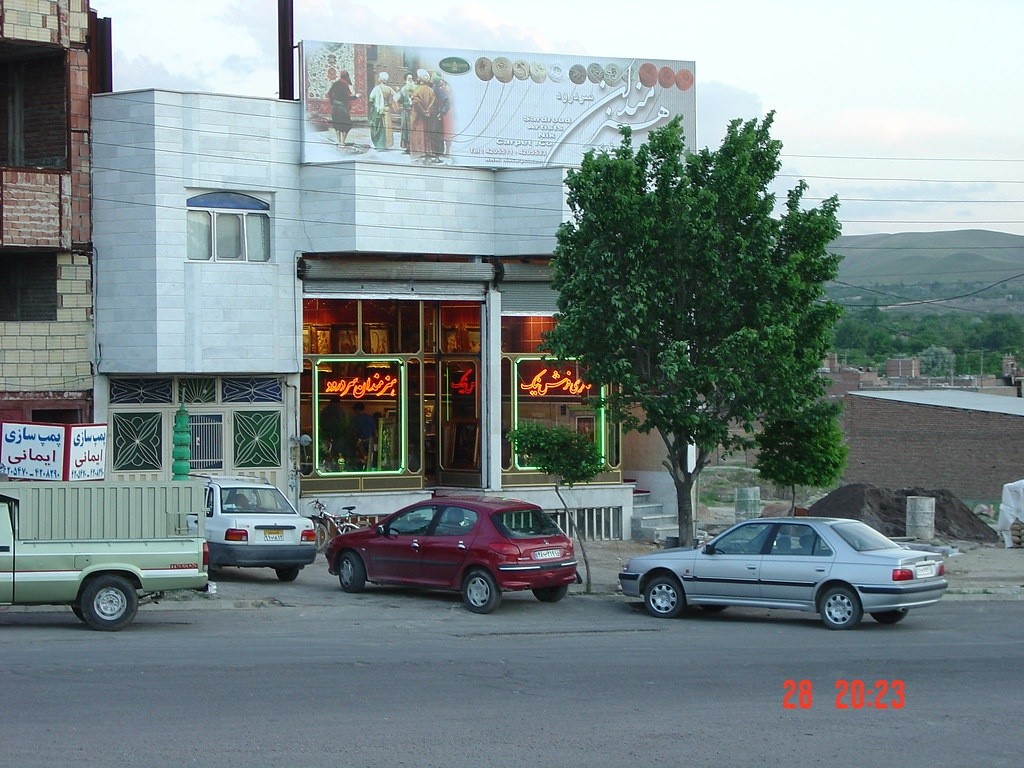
[906,496,935,539]
[735,487,760,524]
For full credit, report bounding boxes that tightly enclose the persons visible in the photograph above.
[327,69,450,165]
[320,381,422,469]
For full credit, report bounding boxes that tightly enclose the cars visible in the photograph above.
[187,474,319,583]
[618,515,950,631]
[323,494,578,616]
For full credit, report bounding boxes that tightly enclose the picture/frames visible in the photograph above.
[377,417,397,470]
[575,416,596,444]
[449,419,480,470]
[367,437,377,471]
[302,323,507,372]
[383,408,397,417]
[414,394,452,424]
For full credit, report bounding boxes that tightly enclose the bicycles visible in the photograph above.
[307,497,375,551]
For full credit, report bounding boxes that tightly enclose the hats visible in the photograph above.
[432,72,441,83]
[379,71,389,83]
[417,69,430,83]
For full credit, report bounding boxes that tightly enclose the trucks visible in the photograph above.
[0,477,217,631]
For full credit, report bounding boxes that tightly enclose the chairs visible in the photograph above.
[775,536,790,553]
[445,508,465,527]
[232,494,249,506]
[800,535,822,555]
